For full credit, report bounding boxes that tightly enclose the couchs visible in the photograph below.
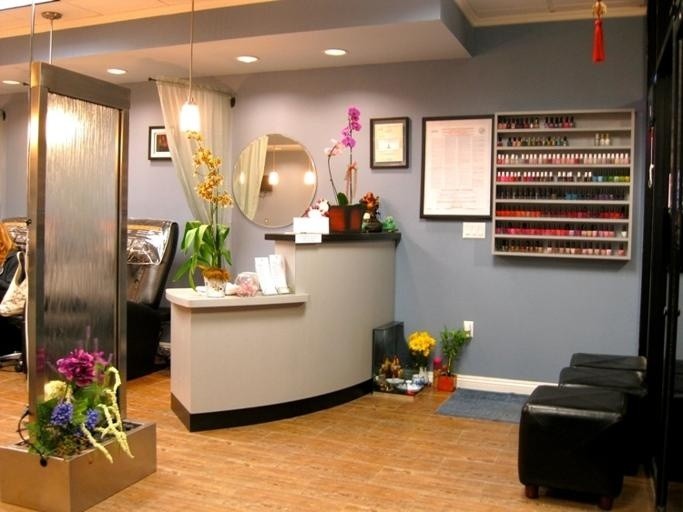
[0,216,177,380]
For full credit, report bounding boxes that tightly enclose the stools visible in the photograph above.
[517,351,646,511]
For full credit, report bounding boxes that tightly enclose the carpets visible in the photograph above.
[433,387,530,423]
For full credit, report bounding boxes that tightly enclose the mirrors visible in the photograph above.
[232,134,318,229]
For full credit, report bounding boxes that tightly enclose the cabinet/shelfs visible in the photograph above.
[490,107,638,262]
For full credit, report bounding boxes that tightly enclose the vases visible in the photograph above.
[0,418,157,511]
[325,201,361,232]
[366,211,383,233]
[201,272,226,298]
[416,366,429,386]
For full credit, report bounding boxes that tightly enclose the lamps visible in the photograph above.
[175,0,201,130]
[268,135,315,186]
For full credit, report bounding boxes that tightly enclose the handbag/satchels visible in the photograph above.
[0,251,26,319]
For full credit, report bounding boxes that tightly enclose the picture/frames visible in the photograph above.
[370,116,410,168]
[147,125,171,160]
[420,113,493,220]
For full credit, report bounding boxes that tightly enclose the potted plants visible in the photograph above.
[437,326,473,392]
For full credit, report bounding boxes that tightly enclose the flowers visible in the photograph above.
[326,104,363,206]
[23,348,135,464]
[358,192,380,215]
[407,330,437,368]
[172,129,233,292]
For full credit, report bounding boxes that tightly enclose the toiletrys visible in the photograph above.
[493,114,630,257]
